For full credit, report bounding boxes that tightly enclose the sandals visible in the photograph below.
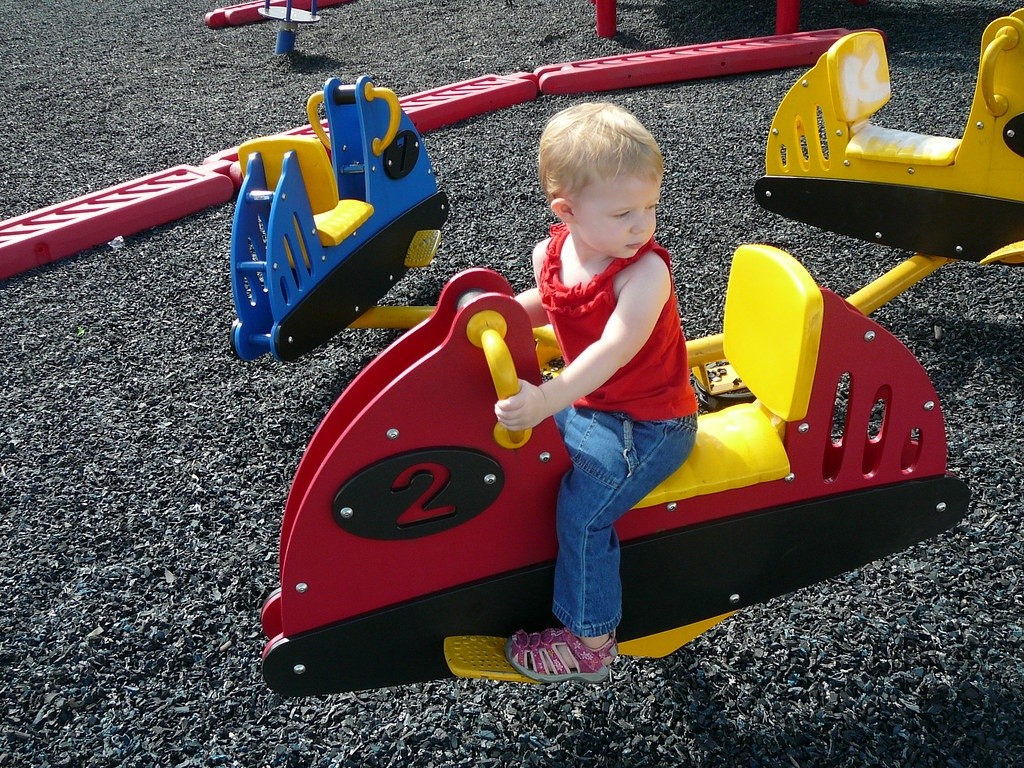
[504,628,618,683]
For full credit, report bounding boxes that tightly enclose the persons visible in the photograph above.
[492,99,700,682]
